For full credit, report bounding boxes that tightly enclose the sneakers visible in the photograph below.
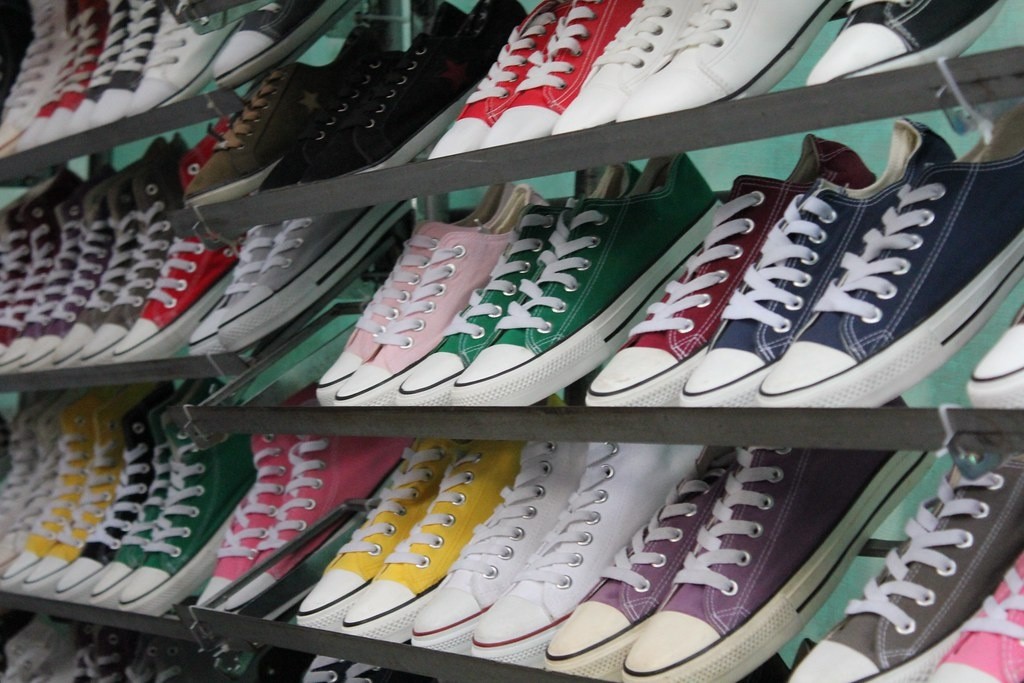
[1,0,1023,683]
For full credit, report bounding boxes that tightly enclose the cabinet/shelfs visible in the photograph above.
[2,0,1023,683]
